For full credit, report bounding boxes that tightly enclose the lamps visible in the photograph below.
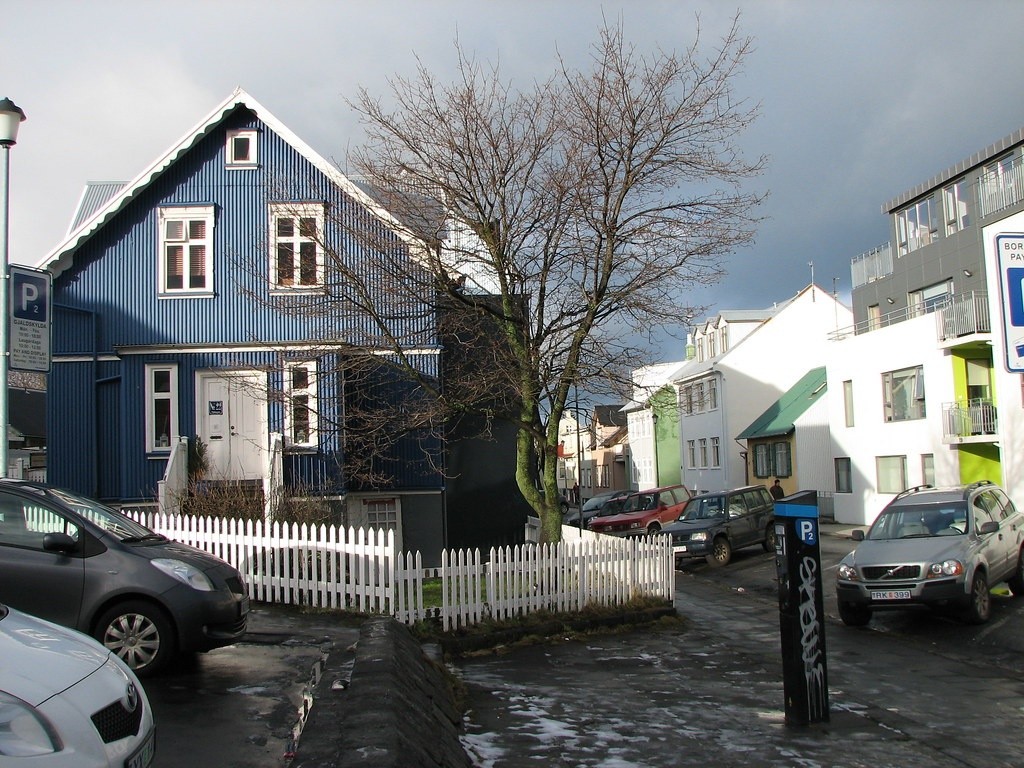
[887,298,895,304]
[963,270,972,276]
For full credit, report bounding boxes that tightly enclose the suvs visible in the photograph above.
[652,485,776,568]
[835,479,1024,626]
[566,490,638,530]
[0,476,251,676]
[602,484,692,544]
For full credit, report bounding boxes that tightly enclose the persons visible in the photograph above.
[573,482,579,499]
[770,479,784,500]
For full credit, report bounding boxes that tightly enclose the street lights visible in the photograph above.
[0,97,27,480]
[573,370,582,528]
[652,414,660,488]
[561,440,565,455]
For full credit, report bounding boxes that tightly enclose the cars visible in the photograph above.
[539,490,570,514]
[589,497,634,531]
[0,603,156,768]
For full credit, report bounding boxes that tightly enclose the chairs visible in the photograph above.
[896,506,990,537]
[706,499,763,518]
